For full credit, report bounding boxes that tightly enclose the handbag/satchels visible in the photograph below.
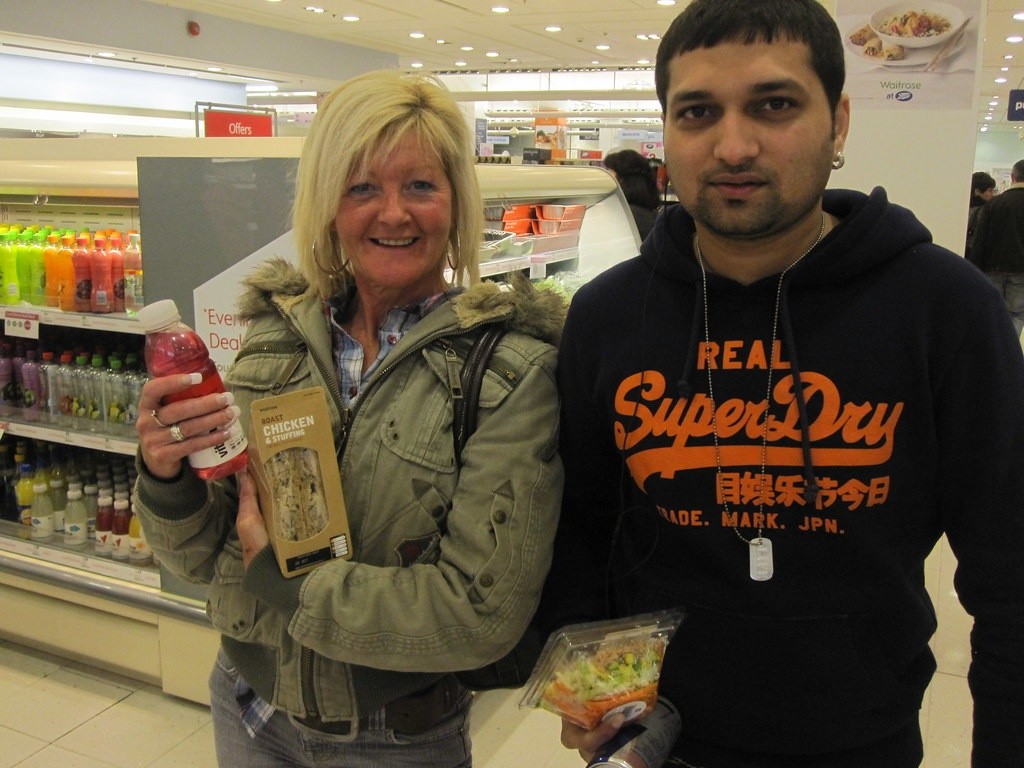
[437,325,564,690]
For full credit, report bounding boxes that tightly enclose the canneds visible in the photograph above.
[586,695,681,768]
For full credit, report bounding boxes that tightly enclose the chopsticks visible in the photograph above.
[923,18,973,72]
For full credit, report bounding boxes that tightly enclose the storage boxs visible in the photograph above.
[242,383,351,578]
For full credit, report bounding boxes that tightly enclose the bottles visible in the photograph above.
[138,299,250,480]
[0,436,161,568]
[0,336,148,438]
[0,221,143,319]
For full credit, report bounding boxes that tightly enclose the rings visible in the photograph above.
[151,409,165,427]
[170,424,185,441]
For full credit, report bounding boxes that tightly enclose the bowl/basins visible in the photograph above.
[519,607,687,729]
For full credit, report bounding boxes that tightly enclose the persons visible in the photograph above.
[133,73,567,768]
[536,126,564,149]
[542,0,1024,768]
[966,160,1024,338]
[603,150,662,243]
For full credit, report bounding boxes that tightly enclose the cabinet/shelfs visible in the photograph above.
[1,131,627,710]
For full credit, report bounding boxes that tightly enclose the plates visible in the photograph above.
[844,20,969,65]
[870,0,965,48]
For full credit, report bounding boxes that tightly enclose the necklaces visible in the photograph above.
[696,213,825,583]
